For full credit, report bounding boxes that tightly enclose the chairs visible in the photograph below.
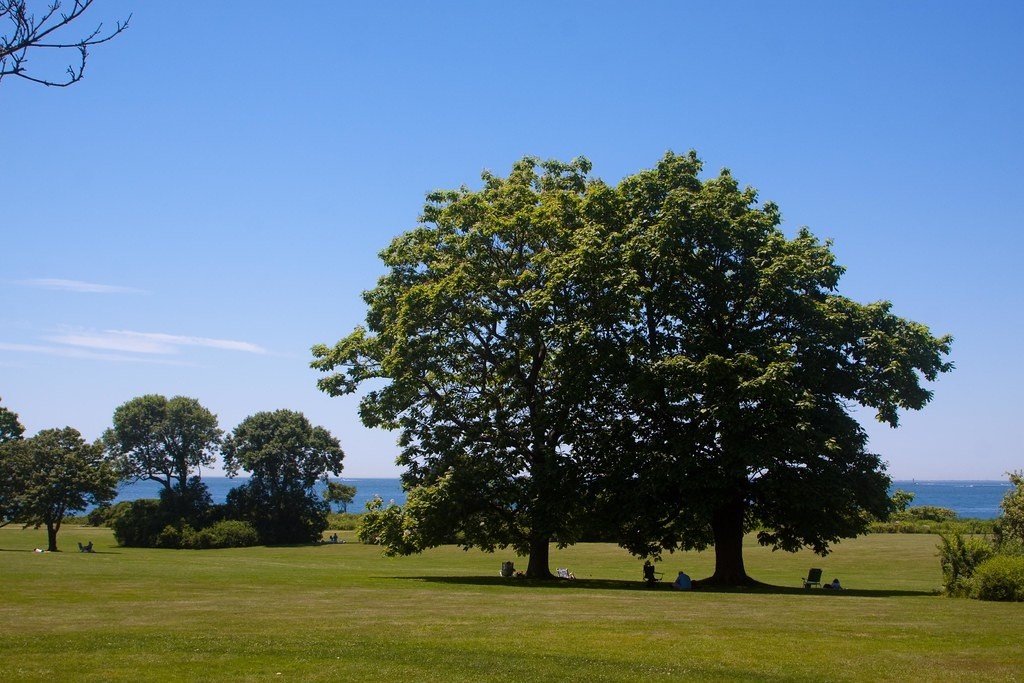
[500,562,515,577]
[802,568,822,589]
[83,544,94,552]
[556,567,575,580]
[643,560,663,583]
[78,543,84,550]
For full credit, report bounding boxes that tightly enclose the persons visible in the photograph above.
[644,561,654,578]
[672,571,691,590]
[502,562,515,576]
[88,541,95,553]
[831,579,842,592]
[329,533,338,543]
[34,548,50,552]
